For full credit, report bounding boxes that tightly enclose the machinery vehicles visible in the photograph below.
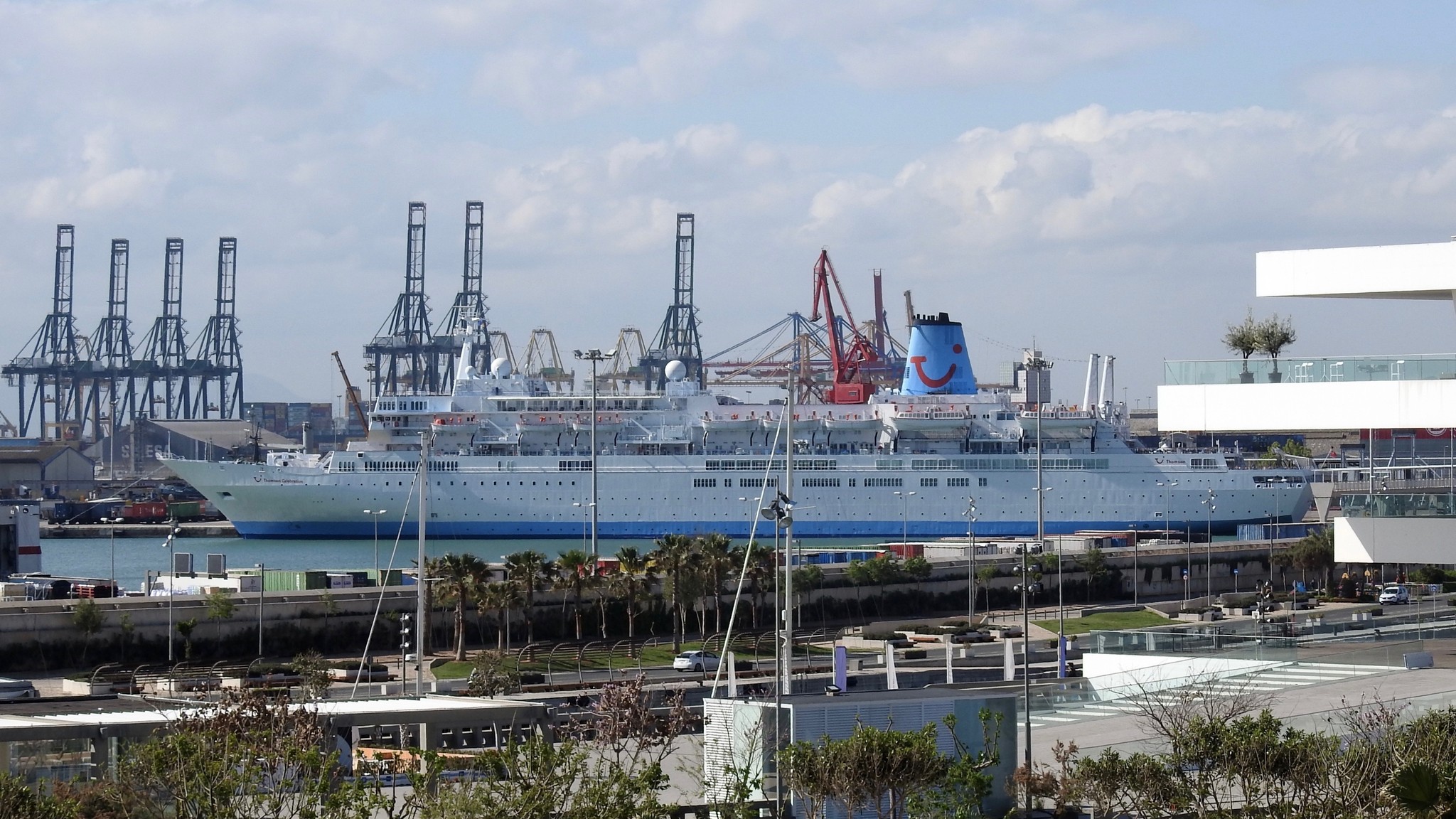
[331,351,369,439]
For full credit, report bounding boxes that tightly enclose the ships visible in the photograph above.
[147,270,1313,569]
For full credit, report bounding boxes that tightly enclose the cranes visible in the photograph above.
[1,224,245,443]
[362,200,917,408]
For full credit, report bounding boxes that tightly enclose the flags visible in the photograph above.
[1331,450,1337,458]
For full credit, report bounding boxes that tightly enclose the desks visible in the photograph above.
[1173,627,1192,652]
[1269,623,1288,649]
[1286,622,1301,647]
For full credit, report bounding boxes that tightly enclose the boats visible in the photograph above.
[428,411,1113,435]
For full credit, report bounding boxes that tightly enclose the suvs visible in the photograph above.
[1379,585,1409,605]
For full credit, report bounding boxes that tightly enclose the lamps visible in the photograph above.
[1321,446,1337,469]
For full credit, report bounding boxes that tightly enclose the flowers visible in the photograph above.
[1221,308,1298,373]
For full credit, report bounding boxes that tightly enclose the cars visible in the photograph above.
[673,650,721,672]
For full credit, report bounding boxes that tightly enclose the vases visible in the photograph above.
[1268,373,1283,383]
[1022,644,1037,653]
[1239,373,1255,383]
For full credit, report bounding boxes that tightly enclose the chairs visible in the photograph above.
[1156,622,1309,653]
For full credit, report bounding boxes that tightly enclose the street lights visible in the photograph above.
[1013,543,1045,819]
[1032,487,1054,552]
[398,612,414,696]
[894,491,917,563]
[1129,524,1138,607]
[570,345,618,578]
[573,502,598,567]
[1267,478,1288,540]
[1110,358,1116,411]
[1264,513,1273,593]
[1201,481,1217,607]
[364,509,389,587]
[966,531,975,617]
[738,496,762,539]
[1255,578,1275,645]
[1024,356,1054,539]
[254,563,265,666]
[962,489,978,626]
[759,475,798,819]
[99,516,125,599]
[1182,519,1191,600]
[160,507,182,673]
[1157,482,1179,545]
[1122,388,1152,409]
[1095,356,1101,412]
[1443,445,1449,478]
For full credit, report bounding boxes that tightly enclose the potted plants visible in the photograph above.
[1207,625,1224,650]
[1362,608,1372,620]
[900,650,927,659]
[1178,593,1327,622]
[1352,609,1363,621]
[62,660,389,694]
[960,642,975,658]
[516,672,545,684]
[725,660,754,671]
[1044,635,1080,650]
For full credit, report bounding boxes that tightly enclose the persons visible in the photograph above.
[1252,462,1256,468]
